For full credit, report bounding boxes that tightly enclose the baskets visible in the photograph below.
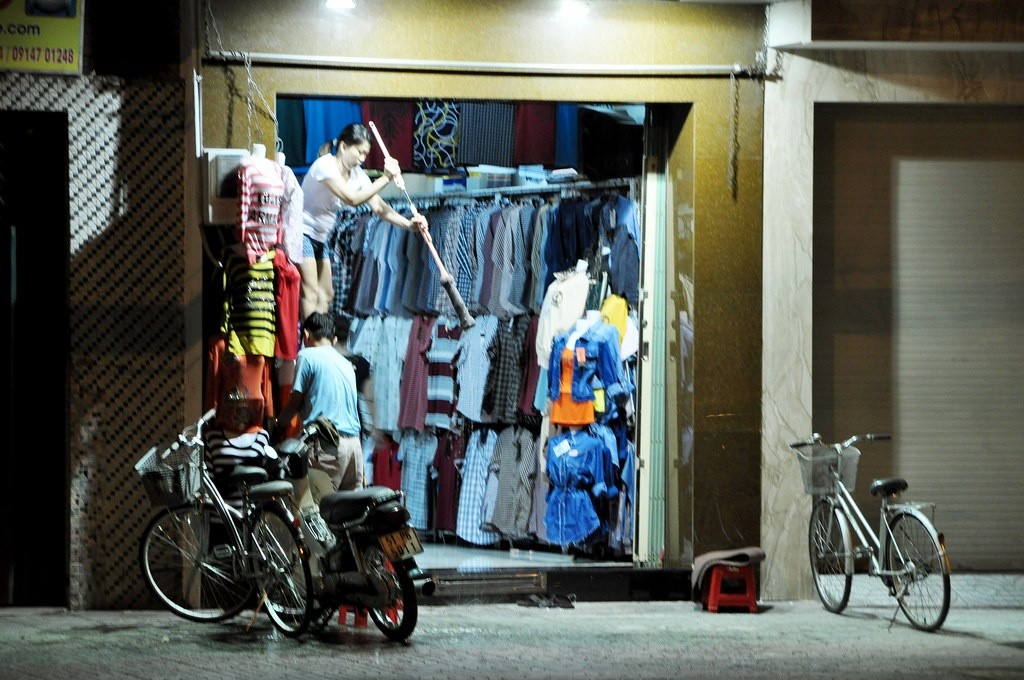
[797,439,861,495]
[135,444,201,507]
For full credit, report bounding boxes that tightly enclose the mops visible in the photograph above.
[366,117,478,332]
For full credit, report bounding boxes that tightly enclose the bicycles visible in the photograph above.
[790,432,951,633]
[132,407,317,639]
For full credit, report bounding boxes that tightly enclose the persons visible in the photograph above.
[535,258,632,544]
[321,311,373,498]
[295,122,430,341]
[205,139,308,443]
[266,309,365,562]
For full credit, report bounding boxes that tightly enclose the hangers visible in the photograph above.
[275,136,285,153]
[338,171,631,215]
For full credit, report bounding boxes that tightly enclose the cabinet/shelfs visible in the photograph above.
[328,179,644,558]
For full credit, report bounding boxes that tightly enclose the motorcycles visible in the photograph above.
[263,402,435,643]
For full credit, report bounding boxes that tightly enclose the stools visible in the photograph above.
[338,558,401,631]
[700,567,758,614]
